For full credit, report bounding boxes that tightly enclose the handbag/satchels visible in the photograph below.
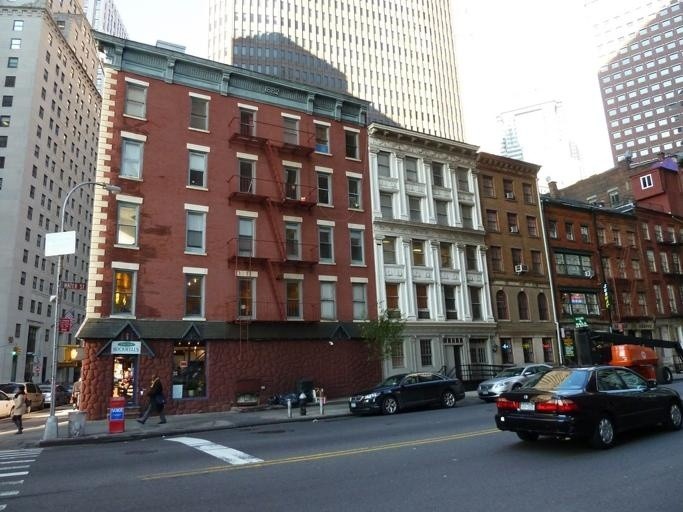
[155,394,167,404]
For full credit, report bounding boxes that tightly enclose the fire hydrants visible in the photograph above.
[299,393,307,415]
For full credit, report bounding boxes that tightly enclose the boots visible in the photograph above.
[17,430,22,434]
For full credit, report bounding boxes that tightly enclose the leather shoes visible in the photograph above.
[157,420,166,424]
[137,419,144,424]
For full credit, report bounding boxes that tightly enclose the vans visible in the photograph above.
[1,382,45,413]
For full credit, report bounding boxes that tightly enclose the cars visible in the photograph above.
[349,372,465,414]
[38,384,68,407]
[477,363,552,402]
[0,390,15,419]
[495,366,682,449]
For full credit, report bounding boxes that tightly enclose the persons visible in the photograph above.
[72,377,81,410]
[12,386,26,434]
[137,375,166,424]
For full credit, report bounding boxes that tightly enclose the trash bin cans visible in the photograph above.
[69,411,87,438]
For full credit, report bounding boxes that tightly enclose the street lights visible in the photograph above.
[42,182,121,441]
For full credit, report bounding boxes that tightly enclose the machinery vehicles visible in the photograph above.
[591,332,683,384]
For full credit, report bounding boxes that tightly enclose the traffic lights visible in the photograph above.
[8,336,14,343]
[12,345,22,356]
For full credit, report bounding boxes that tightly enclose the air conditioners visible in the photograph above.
[584,270,594,277]
[510,226,518,233]
[514,264,528,273]
[505,193,513,199]
